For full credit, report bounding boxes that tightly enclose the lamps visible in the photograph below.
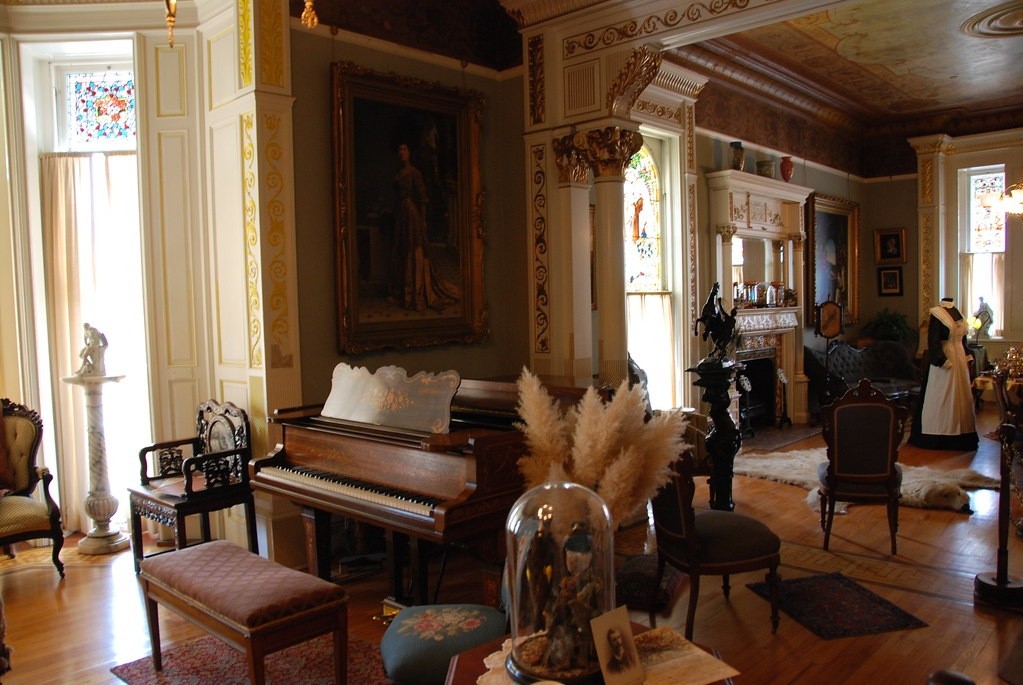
[998,182,1023,215]
[968,311,990,348]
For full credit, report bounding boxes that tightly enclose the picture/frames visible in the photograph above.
[330,61,490,354]
[804,192,862,326]
[874,228,907,264]
[877,266,903,296]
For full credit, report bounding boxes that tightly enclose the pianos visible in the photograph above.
[249,362,651,625]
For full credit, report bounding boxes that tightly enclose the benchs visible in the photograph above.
[139,540,350,685]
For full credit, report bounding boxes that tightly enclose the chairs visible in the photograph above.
[647,451,780,644]
[0,398,66,579]
[126,399,259,575]
[816,378,909,555]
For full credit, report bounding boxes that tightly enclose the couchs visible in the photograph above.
[804,339,923,428]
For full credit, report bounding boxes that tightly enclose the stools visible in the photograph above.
[911,386,922,417]
[380,604,506,684]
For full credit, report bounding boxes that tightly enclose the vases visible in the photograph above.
[780,156,794,183]
[728,141,745,171]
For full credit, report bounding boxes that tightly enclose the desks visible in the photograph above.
[971,372,1023,413]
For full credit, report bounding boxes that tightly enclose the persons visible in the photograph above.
[607,629,633,671]
[718,297,737,358]
[907,298,979,447]
[75,323,105,374]
[979,297,990,334]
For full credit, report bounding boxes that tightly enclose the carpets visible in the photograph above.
[110,631,387,685]
[614,518,689,617]
[733,447,946,490]
[746,572,930,641]
